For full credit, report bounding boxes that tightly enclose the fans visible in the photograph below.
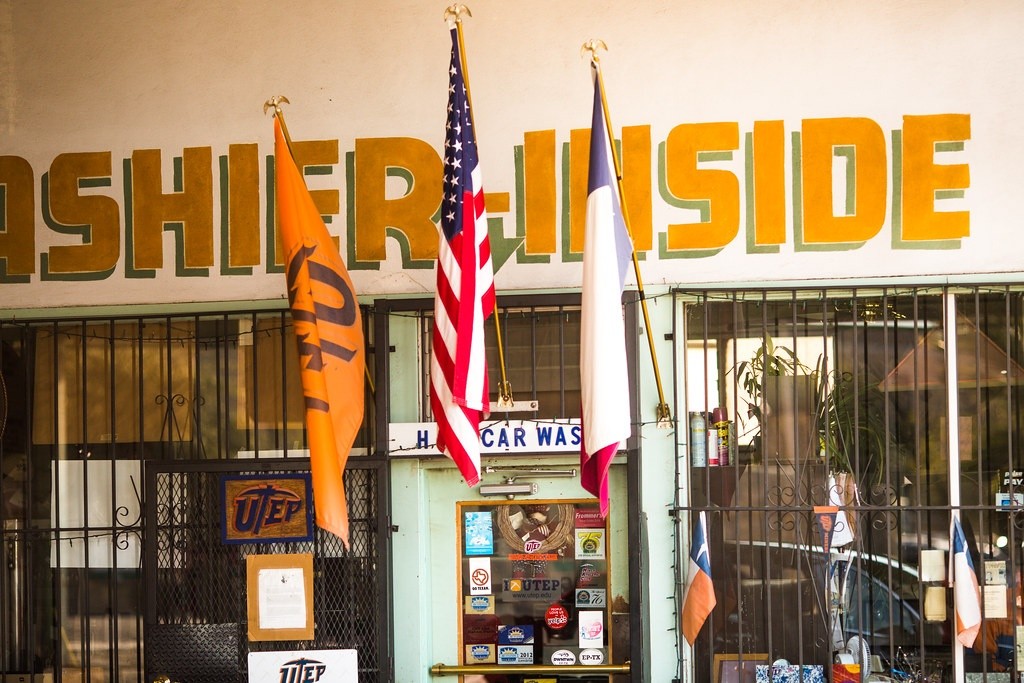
[835,636,872,680]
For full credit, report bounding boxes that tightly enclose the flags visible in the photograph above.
[681,514,717,646]
[954,517,982,648]
[430,26,495,488]
[579,75,633,517]
[274,120,364,553]
